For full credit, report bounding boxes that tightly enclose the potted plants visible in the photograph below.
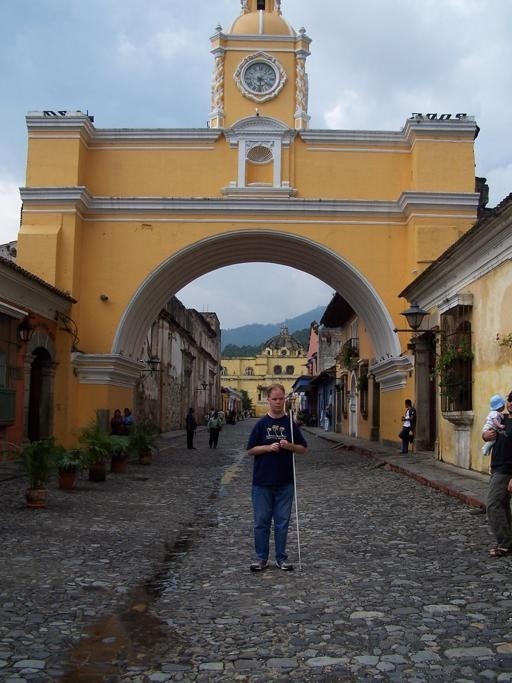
[0,429,65,509]
[76,427,113,481]
[106,434,133,473]
[57,445,82,490]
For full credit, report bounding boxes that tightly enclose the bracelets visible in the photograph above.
[290,443,295,452]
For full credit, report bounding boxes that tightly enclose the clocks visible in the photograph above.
[232,51,287,104]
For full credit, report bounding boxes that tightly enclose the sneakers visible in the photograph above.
[274,559,294,571]
[249,559,271,571]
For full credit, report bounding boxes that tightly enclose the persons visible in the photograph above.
[110,407,127,435]
[185,407,198,449]
[481,390,511,557]
[397,398,416,454]
[204,406,251,426]
[480,394,506,455]
[322,405,331,431]
[207,410,223,449]
[245,382,309,571]
[122,407,135,425]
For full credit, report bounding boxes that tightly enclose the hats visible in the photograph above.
[487,393,507,411]
